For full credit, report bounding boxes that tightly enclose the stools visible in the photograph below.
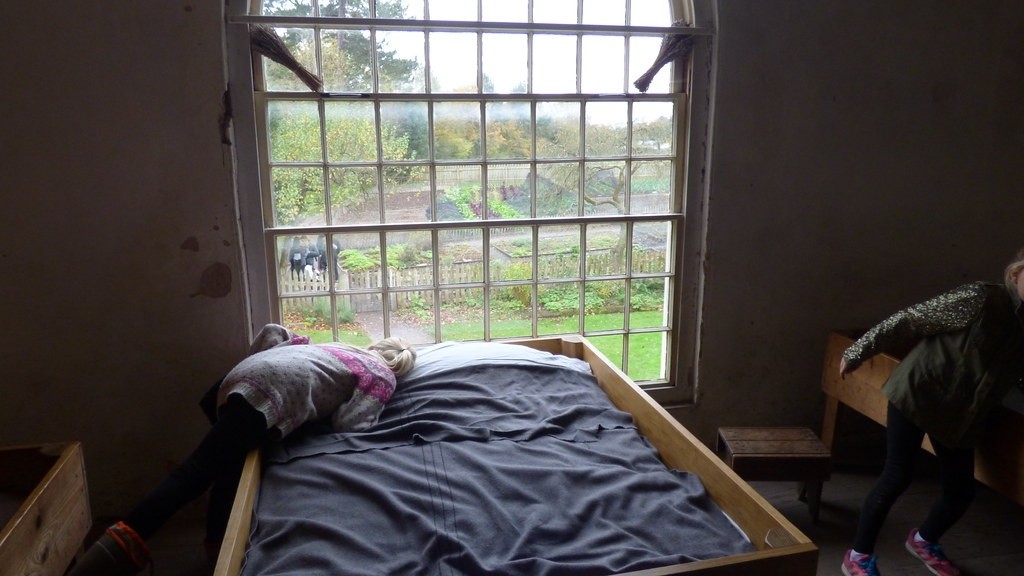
[716,423,827,527]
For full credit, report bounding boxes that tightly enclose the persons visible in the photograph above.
[840,256,1024,576]
[67,323,416,576]
[289,235,340,290]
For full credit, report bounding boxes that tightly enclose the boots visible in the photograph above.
[65,522,154,576]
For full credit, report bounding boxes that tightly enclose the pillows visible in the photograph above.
[399,338,592,379]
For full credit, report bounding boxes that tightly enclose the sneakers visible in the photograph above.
[841,549,881,576]
[904,529,961,576]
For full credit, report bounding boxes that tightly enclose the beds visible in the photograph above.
[814,328,1023,509]
[207,333,821,575]
